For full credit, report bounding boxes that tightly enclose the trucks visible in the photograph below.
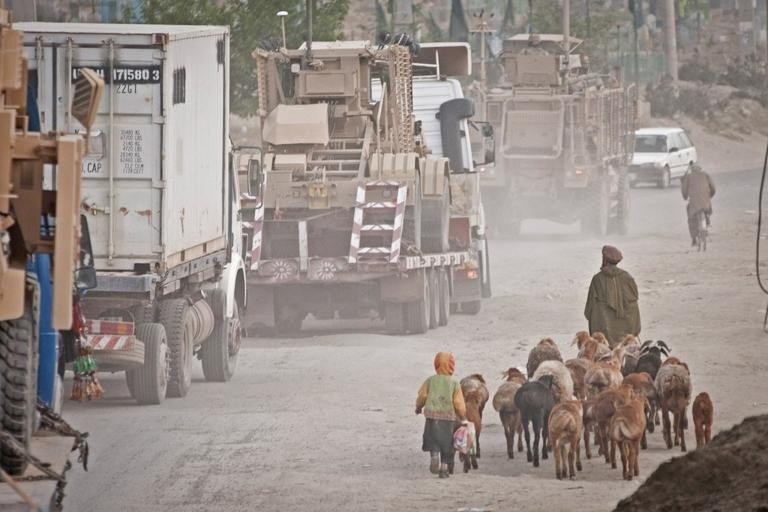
[469,32,637,253]
[231,27,492,340]
[13,17,248,402]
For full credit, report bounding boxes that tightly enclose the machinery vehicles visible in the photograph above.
[232,36,451,261]
[0,1,98,512]
[476,1,603,154]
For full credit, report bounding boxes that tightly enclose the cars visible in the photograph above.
[630,125,696,191]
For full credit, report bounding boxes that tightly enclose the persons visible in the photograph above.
[680,160,715,246]
[413,351,468,478]
[584,245,641,347]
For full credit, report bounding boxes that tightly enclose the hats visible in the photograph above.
[602,245,623,263]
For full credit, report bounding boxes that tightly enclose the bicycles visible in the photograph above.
[690,205,712,252]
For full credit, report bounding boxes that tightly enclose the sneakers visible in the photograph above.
[430,456,440,475]
[440,469,449,477]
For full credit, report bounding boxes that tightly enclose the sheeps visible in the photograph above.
[694,390,714,448]
[459,374,489,471]
[492,330,692,481]
